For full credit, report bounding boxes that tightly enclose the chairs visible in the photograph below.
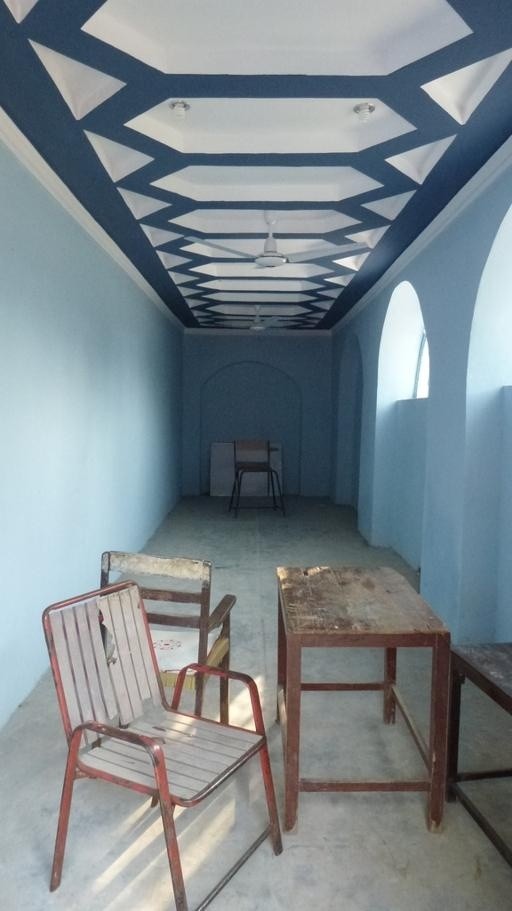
[228,438,286,519]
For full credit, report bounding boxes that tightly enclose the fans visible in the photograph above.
[213,305,302,334]
[183,215,369,273]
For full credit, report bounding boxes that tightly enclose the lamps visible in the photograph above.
[353,102,375,124]
[170,99,193,120]
